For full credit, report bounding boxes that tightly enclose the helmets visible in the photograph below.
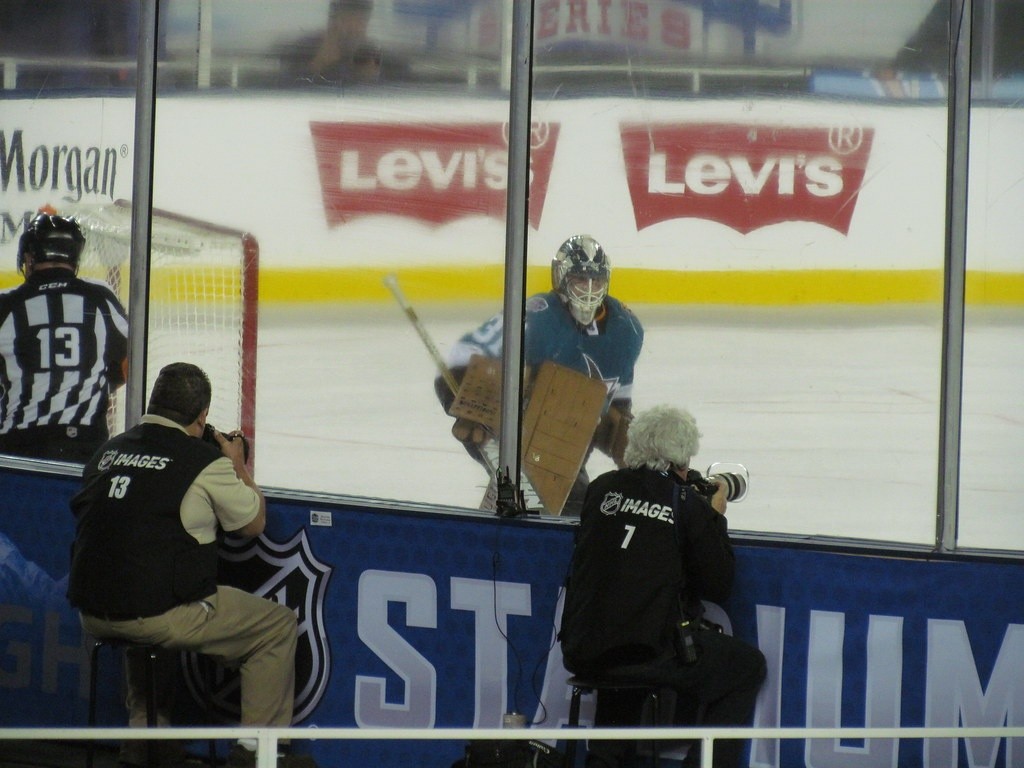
[17,215,84,266]
[551,234,610,325]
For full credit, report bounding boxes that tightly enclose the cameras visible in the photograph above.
[202,424,233,450]
[686,469,747,502]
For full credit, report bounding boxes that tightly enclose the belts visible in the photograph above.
[83,607,168,621]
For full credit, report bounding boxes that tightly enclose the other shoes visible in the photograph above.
[221,745,317,767]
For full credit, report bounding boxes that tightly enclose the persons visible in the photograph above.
[560,404,768,768]
[434,235,644,520]
[0,210,130,466]
[67,361,299,768]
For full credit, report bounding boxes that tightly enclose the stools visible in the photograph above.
[566,676,663,768]
[84,638,216,768]
